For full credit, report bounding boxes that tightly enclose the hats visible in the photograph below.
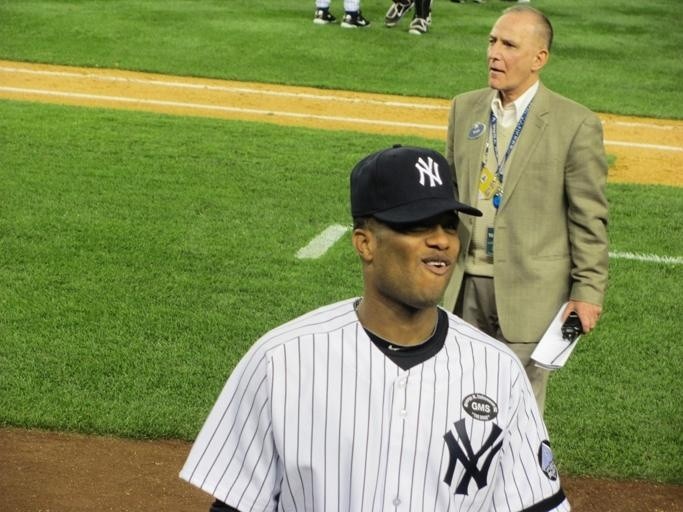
[350,143,483,222]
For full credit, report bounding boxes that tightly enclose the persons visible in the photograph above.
[176,143,571,512]
[441,5,611,417]
[384,0,432,36]
[312,0,372,29]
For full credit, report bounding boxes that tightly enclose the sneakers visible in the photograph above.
[384,0,412,27]
[342,12,371,27]
[410,13,432,34]
[312,10,336,24]
